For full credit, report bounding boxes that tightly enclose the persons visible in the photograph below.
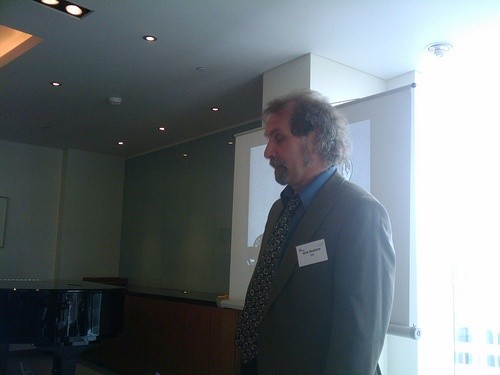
[234,86,396,375]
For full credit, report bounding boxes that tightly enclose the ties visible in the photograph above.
[236,194,304,368]
